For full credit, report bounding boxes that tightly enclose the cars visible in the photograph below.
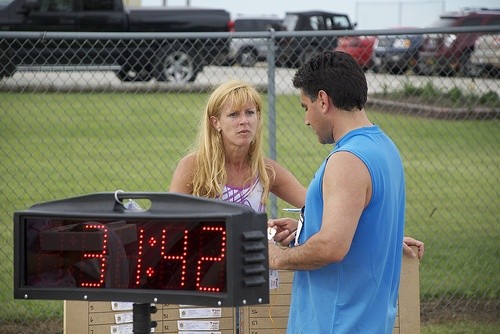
[336,36,373,70]
[474,32,500,75]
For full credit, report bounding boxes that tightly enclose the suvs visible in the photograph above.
[421,11,497,77]
[283,12,349,64]
[231,16,283,67]
[373,28,423,75]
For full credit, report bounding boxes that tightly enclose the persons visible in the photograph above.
[169,81,424,258]
[267,50,405,334]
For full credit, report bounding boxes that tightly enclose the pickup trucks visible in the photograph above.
[0,0,231,80]
